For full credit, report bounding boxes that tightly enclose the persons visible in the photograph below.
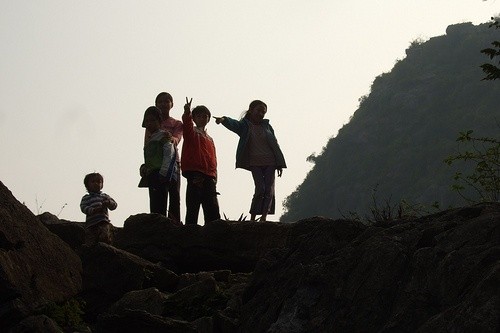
[146,92,183,222]
[81,172,117,246]
[138,106,175,216]
[213,99,287,221]
[182,95,221,224]
